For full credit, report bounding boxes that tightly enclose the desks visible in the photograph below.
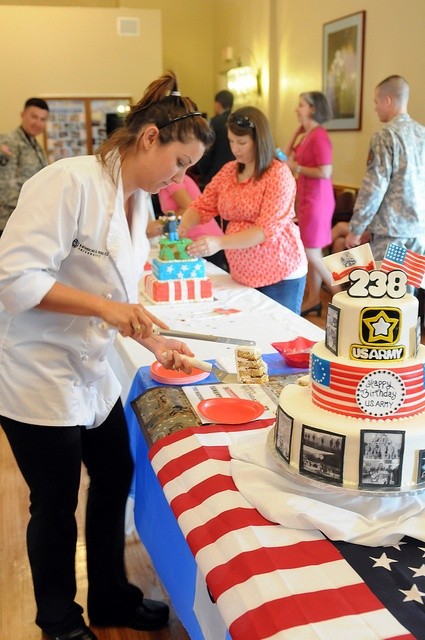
[115,255,425,640]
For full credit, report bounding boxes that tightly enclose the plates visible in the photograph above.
[271,336,318,368]
[197,397,266,425]
[150,360,210,385]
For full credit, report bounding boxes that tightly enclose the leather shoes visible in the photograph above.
[43,620,98,638]
[86,596,170,630]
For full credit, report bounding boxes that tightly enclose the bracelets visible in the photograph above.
[296,164,302,173]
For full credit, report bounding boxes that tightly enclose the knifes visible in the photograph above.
[98,321,257,347]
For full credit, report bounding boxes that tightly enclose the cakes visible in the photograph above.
[142,209,213,303]
[234,344,269,384]
[272,239,425,494]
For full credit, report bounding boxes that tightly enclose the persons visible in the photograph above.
[327,221,372,254]
[344,73,425,300]
[1,97,50,236]
[280,91,346,320]
[193,89,236,190]
[1,69,217,639]
[174,106,309,318]
[156,173,231,276]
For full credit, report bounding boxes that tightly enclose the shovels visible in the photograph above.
[161,351,242,383]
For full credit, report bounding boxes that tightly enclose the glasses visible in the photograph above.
[225,112,256,130]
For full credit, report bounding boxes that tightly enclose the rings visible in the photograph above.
[133,324,140,331]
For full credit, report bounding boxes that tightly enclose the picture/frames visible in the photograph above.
[321,10,366,132]
